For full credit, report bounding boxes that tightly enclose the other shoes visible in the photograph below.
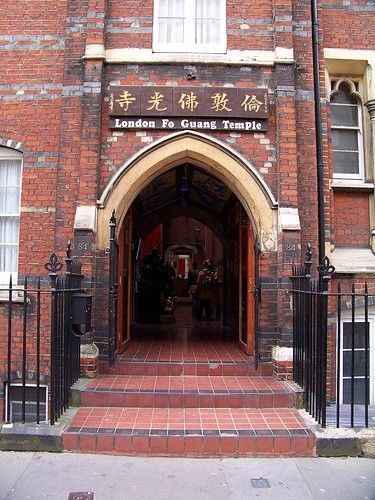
[195,316,201,320]
[191,312,195,317]
[203,316,211,320]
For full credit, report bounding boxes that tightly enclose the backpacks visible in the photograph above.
[199,269,214,290]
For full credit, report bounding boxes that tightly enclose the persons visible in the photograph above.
[137,246,221,324]
